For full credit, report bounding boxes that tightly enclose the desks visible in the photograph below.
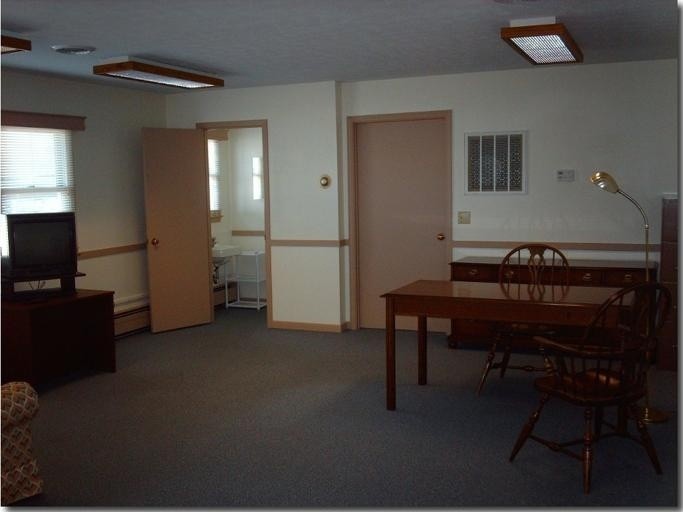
[1,293,115,393]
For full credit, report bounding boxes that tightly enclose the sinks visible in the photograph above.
[212,245,241,266]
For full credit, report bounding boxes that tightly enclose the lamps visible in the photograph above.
[589,170,653,281]
[85,55,226,92]
[499,17,584,66]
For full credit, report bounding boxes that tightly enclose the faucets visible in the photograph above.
[212,237,218,247]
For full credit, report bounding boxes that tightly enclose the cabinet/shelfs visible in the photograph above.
[225,249,268,312]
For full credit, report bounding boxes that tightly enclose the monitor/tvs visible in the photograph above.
[1,212,77,277]
